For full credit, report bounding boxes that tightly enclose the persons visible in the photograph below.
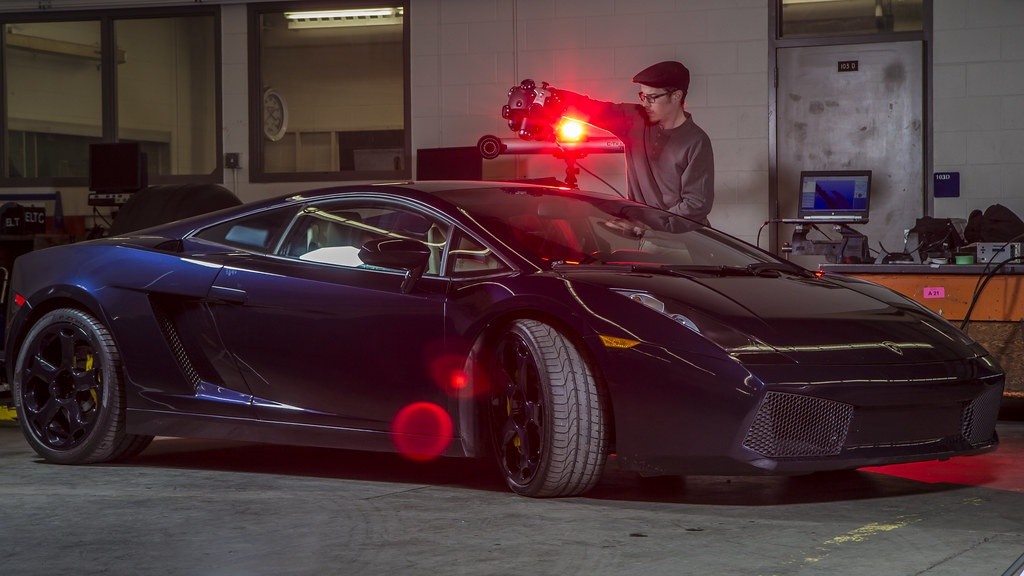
[547,61,714,229]
[525,188,704,240]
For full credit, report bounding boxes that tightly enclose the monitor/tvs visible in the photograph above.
[417,146,482,182]
[88,142,139,191]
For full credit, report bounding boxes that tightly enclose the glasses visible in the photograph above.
[638,92,672,103]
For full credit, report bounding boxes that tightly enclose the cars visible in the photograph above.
[5,178,1006,498]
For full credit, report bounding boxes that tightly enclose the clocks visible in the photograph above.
[263,88,289,141]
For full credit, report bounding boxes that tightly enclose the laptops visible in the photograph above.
[783,170,873,222]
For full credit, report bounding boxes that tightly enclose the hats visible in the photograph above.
[631,60,690,90]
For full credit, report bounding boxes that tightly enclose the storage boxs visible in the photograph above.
[6,207,46,235]
